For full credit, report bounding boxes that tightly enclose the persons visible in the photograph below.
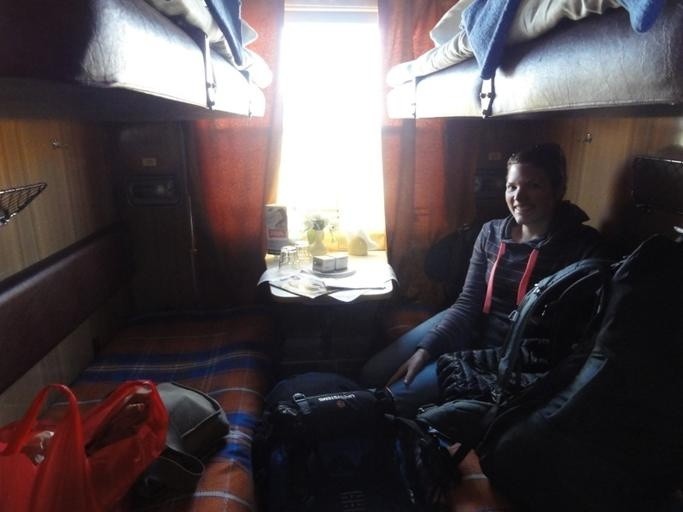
[346,136,599,419]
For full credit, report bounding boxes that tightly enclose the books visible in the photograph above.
[267,271,343,300]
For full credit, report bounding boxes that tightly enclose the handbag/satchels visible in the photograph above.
[129,379,230,497]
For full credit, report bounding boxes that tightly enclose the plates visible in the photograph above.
[310,267,356,277]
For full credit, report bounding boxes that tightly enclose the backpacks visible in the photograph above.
[258,370,447,512]
[472,225,683,509]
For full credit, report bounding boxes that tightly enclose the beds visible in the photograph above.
[388,302,682,510]
[0,1,271,118]
[386,2,683,122]
[0,308,270,512]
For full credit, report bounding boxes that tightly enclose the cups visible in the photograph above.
[277,241,311,276]
